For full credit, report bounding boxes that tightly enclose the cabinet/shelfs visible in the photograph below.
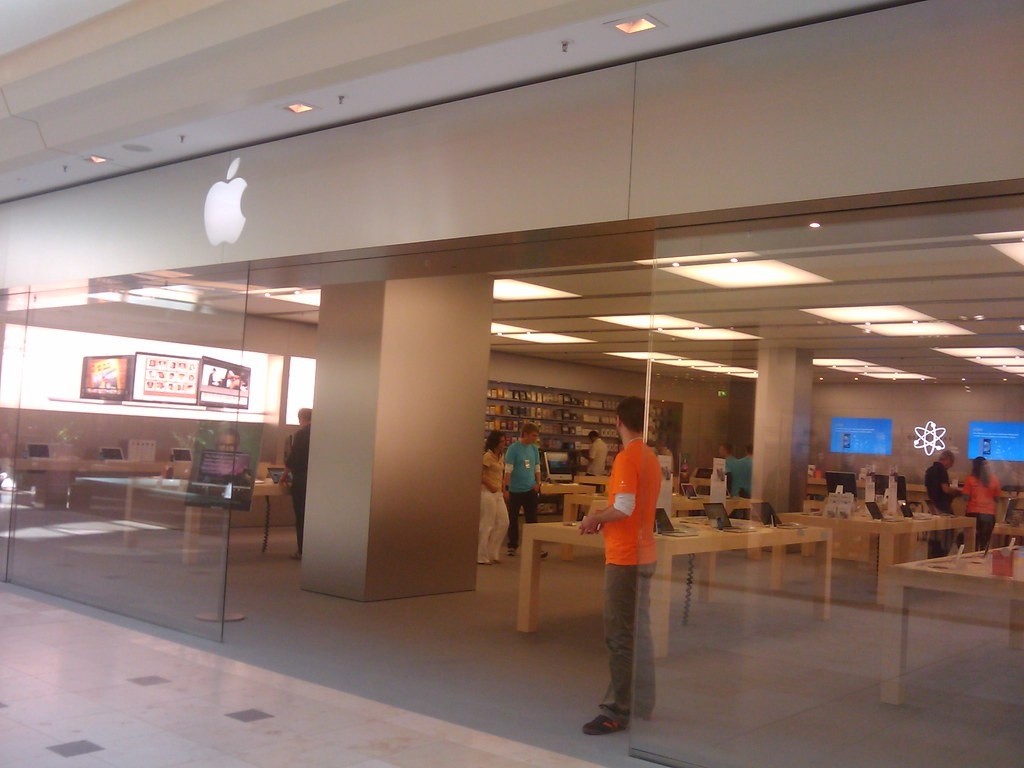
[483,379,681,473]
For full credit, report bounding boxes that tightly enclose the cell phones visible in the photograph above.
[843,433,851,448]
[983,438,990,455]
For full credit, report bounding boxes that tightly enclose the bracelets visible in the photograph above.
[537,481,542,487]
[281,472,288,477]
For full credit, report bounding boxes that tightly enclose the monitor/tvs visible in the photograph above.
[544,451,574,480]
[1003,497,1017,522]
[80,351,251,412]
[824,472,906,505]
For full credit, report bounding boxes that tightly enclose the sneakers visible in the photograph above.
[583,715,625,734]
[540,550,548,558]
[506,548,516,556]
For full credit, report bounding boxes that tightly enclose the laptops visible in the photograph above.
[654,483,808,537]
[864,502,932,523]
[26,442,56,461]
[266,467,292,485]
[170,448,193,464]
[100,446,128,463]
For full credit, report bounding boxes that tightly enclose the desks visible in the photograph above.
[1,455,1024,708]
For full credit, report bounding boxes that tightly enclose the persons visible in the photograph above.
[477,431,510,565]
[215,427,241,453]
[962,456,1002,559]
[577,430,608,522]
[924,450,962,560]
[681,458,689,472]
[718,440,754,520]
[503,423,548,558]
[579,396,662,735]
[207,367,247,390]
[278,409,312,560]
[653,438,672,456]
